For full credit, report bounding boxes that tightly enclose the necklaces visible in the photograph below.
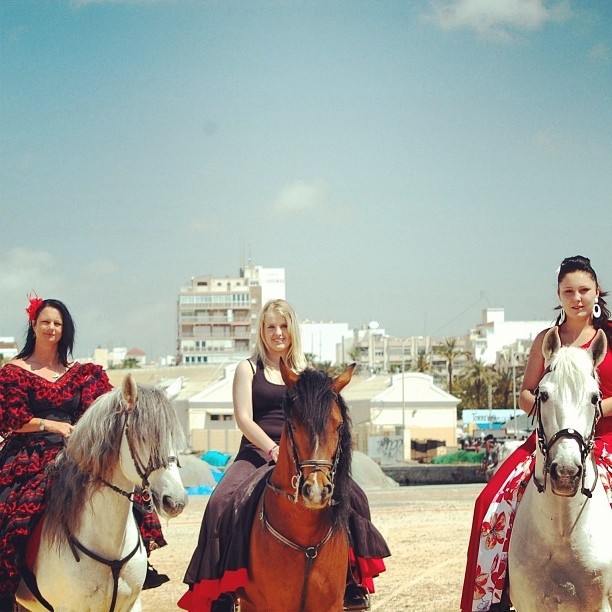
[53,372,59,378]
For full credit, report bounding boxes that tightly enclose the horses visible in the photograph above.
[11,371,190,611]
[222,355,357,612]
[502,324,612,612]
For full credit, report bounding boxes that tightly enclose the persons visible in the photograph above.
[1,290,171,612]
[461,254,612,612]
[177,298,390,611]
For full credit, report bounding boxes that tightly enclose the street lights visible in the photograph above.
[382,335,423,461]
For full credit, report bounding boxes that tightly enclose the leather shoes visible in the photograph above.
[142,566,169,589]
[344,583,366,606]
[211,595,232,610]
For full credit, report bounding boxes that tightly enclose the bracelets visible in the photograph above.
[40,419,46,432]
[269,446,279,457]
